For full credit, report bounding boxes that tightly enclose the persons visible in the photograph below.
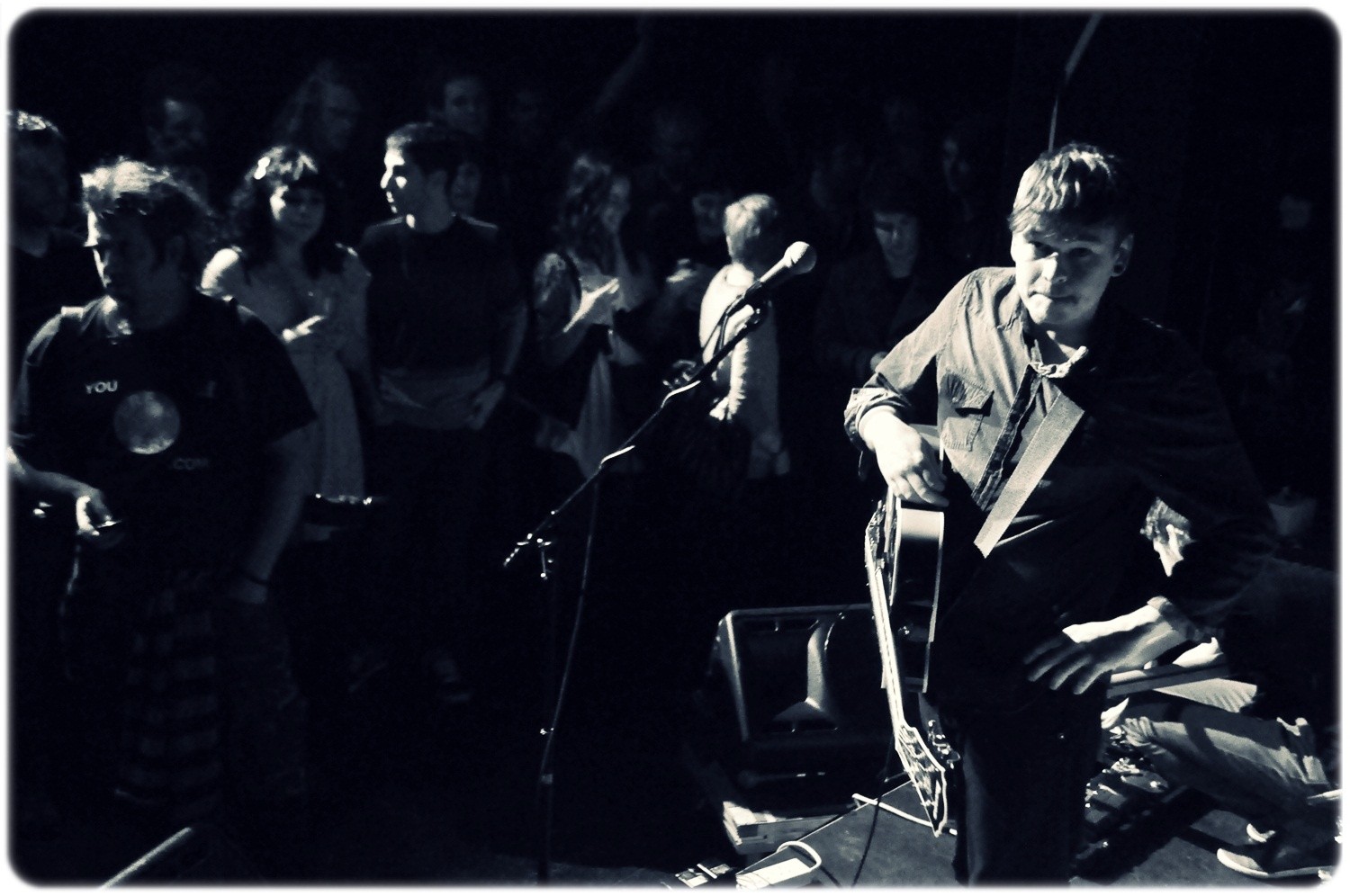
[7,62,1341,886]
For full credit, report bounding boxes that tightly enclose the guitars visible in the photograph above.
[863,421,968,842]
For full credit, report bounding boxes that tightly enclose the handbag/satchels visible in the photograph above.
[507,250,603,421]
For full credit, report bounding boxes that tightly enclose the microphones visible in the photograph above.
[302,492,394,525]
[726,240,818,319]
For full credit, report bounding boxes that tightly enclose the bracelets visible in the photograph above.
[243,569,268,587]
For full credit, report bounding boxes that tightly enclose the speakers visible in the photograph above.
[702,604,897,760]
